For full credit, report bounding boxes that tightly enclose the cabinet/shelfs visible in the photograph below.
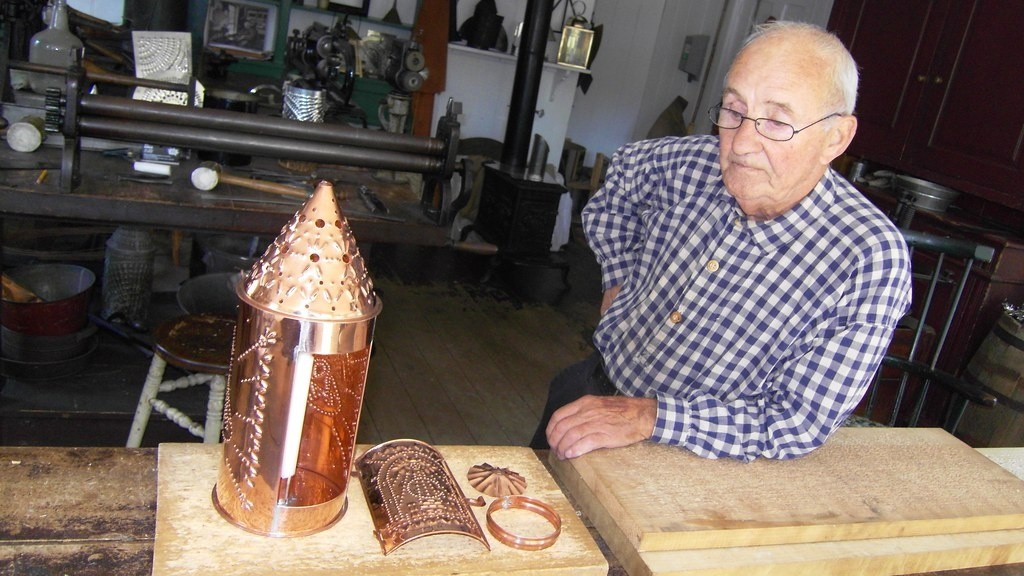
[856,183,1024,427]
[827,0,1024,212]
[188,0,422,130]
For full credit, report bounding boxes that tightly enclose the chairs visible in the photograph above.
[866,354,997,434]
[863,228,995,429]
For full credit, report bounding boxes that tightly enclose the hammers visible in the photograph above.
[190,160,315,200]
[0,115,46,152]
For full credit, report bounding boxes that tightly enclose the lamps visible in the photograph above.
[557,16,594,70]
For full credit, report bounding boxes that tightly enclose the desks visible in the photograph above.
[1,140,498,425]
[0,446,1024,576]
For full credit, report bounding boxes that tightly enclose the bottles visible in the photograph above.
[99,225,155,324]
[29,0,85,96]
[847,153,865,183]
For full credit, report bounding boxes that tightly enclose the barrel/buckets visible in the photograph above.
[952,307,1024,447]
[203,235,272,273]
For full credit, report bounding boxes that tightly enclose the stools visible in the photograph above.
[126,310,237,448]
[206,89,258,164]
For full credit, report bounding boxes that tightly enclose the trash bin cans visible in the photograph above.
[558,141,587,182]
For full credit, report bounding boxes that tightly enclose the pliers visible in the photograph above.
[357,184,390,215]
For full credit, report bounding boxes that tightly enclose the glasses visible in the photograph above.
[707,102,842,141]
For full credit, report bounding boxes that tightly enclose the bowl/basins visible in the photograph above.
[0,262,95,338]
[177,275,239,320]
[892,174,959,213]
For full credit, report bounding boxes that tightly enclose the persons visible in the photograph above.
[530,16,914,464]
